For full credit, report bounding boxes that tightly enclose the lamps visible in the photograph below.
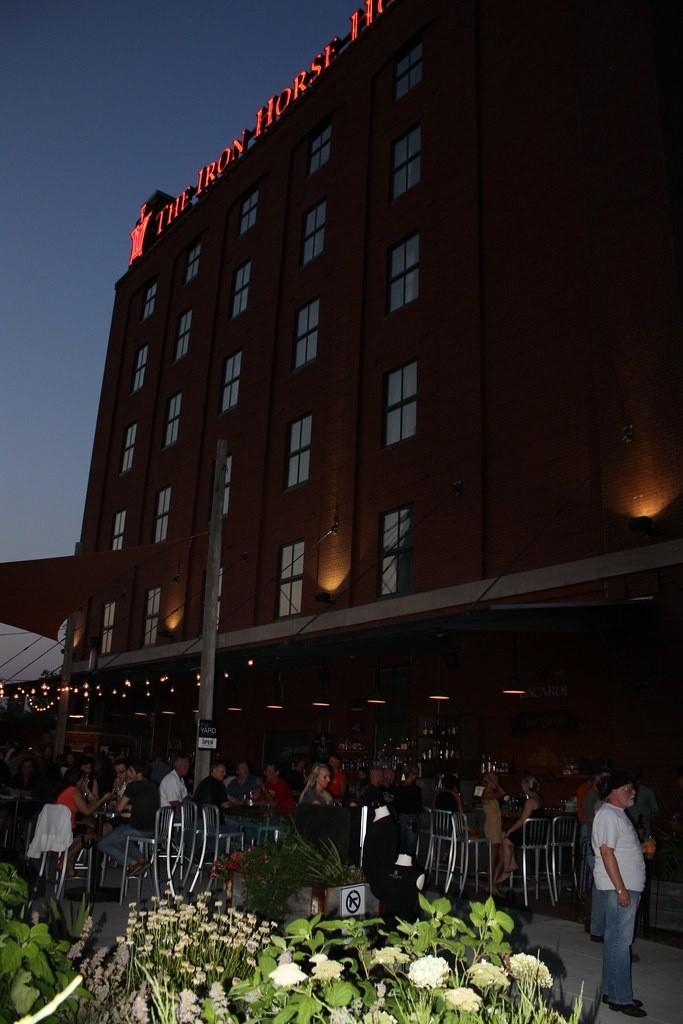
[158,629,177,640]
[627,516,658,542]
[315,592,336,607]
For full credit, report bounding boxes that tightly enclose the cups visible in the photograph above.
[116,777,123,790]
[645,839,655,860]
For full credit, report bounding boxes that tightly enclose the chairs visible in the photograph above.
[0,794,283,909]
[409,807,581,910]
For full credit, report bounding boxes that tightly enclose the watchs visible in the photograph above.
[261,786,266,791]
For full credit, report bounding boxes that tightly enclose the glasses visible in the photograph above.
[113,769,128,776]
[618,787,633,793]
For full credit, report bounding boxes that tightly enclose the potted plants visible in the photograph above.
[249,808,367,919]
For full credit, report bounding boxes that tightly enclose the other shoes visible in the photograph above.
[609,1002,648,1017]
[602,994,643,1006]
[442,850,450,862]
[133,861,150,875]
[590,934,604,943]
[485,886,496,896]
[56,853,74,876]
[493,888,505,898]
[127,862,142,876]
[632,954,640,962]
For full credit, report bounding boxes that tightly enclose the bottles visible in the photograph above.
[422,721,460,759]
[112,791,118,804]
[344,737,416,771]
[248,789,254,807]
[481,753,515,775]
[635,814,645,843]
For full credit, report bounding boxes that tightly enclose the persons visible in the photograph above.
[431,774,463,860]
[576,767,659,961]
[56,770,113,877]
[0,739,423,872]
[590,772,648,1018]
[504,772,544,873]
[482,774,512,899]
[671,763,683,835]
[298,763,335,806]
[96,760,159,877]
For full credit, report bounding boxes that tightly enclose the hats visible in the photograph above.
[601,768,633,800]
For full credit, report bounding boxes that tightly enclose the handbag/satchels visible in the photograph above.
[460,824,479,838]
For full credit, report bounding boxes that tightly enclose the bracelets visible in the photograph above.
[616,889,626,894]
